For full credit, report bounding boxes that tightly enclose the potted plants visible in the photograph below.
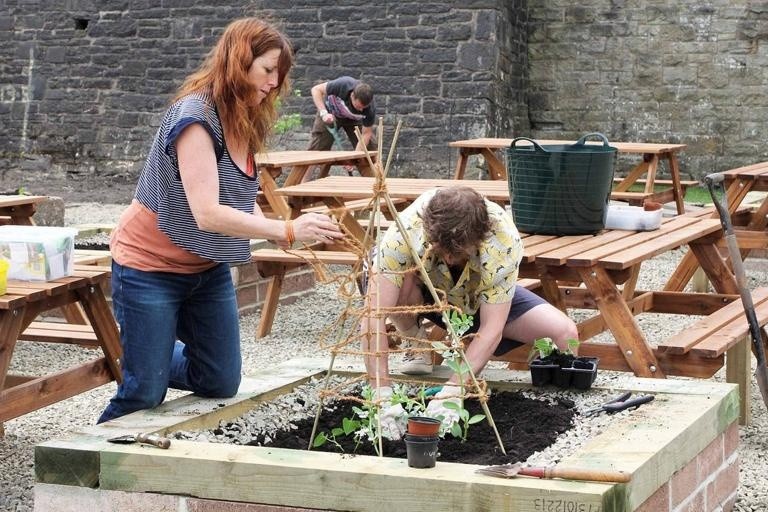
[382,375,452,439]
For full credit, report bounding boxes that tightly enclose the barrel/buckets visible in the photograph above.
[502,131,618,234]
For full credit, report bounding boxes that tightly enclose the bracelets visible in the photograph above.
[319,109,328,120]
[286,218,296,251]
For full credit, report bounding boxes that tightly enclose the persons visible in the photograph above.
[306,74,378,151]
[358,185,581,441]
[97,17,345,426]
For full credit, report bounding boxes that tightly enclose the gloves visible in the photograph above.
[421,380,466,442]
[366,385,412,441]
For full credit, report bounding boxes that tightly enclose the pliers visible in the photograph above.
[586,390,655,418]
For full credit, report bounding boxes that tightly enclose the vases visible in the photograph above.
[402,430,444,470]
[521,330,603,391]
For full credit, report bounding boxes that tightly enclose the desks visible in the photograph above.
[0,190,129,445]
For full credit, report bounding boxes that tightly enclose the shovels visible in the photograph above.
[323,115,370,219]
[474,465,632,483]
[107,433,171,449]
[704,173,768,409]
